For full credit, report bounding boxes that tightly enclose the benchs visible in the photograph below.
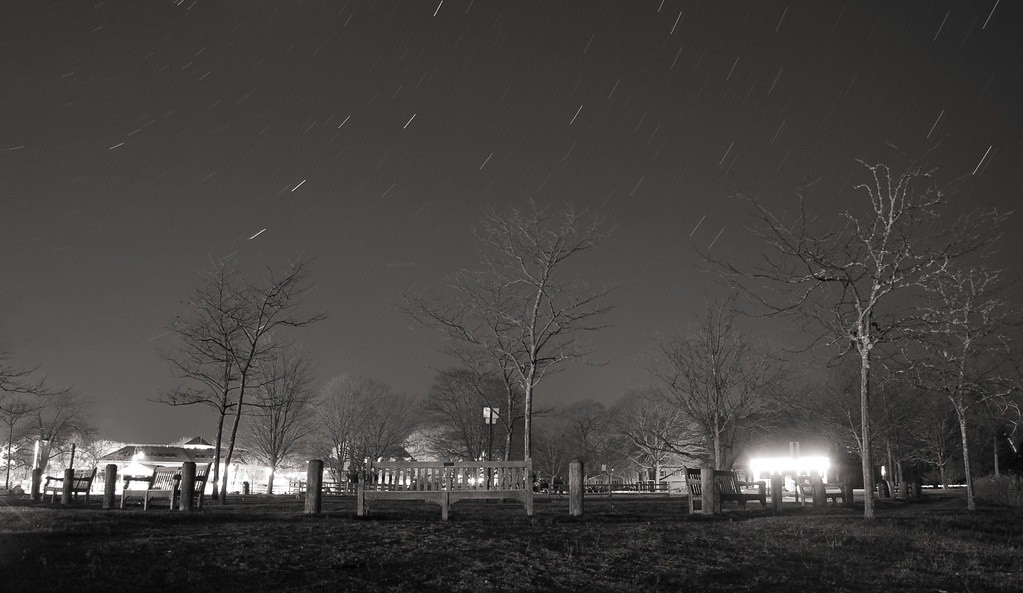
[119,461,213,510]
[682,465,767,514]
[358,456,535,523]
[41,466,98,504]
[798,480,846,506]
